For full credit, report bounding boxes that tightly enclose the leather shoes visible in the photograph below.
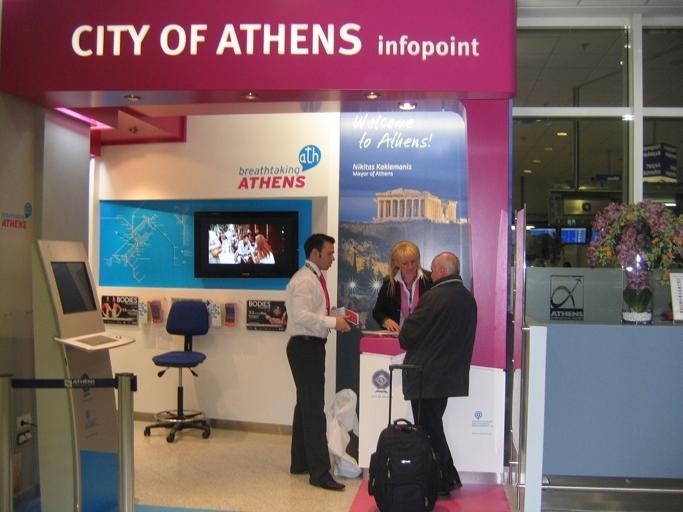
[438,478,462,499]
[321,479,344,491]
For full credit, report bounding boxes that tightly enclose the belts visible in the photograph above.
[292,335,326,343]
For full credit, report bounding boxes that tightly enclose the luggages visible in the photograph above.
[368,364,442,511]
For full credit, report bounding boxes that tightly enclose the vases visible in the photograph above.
[618,265,657,323]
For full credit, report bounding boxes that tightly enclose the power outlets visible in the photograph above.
[14,410,32,433]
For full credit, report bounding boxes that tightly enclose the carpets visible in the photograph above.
[343,470,512,511]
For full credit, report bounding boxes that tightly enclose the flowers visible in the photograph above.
[585,199,683,316]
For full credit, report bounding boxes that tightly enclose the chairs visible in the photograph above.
[141,299,213,445]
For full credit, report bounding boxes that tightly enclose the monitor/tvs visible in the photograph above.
[589,227,600,245]
[50,261,98,315]
[194,211,299,278]
[559,226,588,245]
[531,226,557,242]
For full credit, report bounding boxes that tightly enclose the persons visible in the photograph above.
[286,233,351,489]
[372,241,434,332]
[102,297,121,318]
[399,252,476,500]
[208,223,275,264]
